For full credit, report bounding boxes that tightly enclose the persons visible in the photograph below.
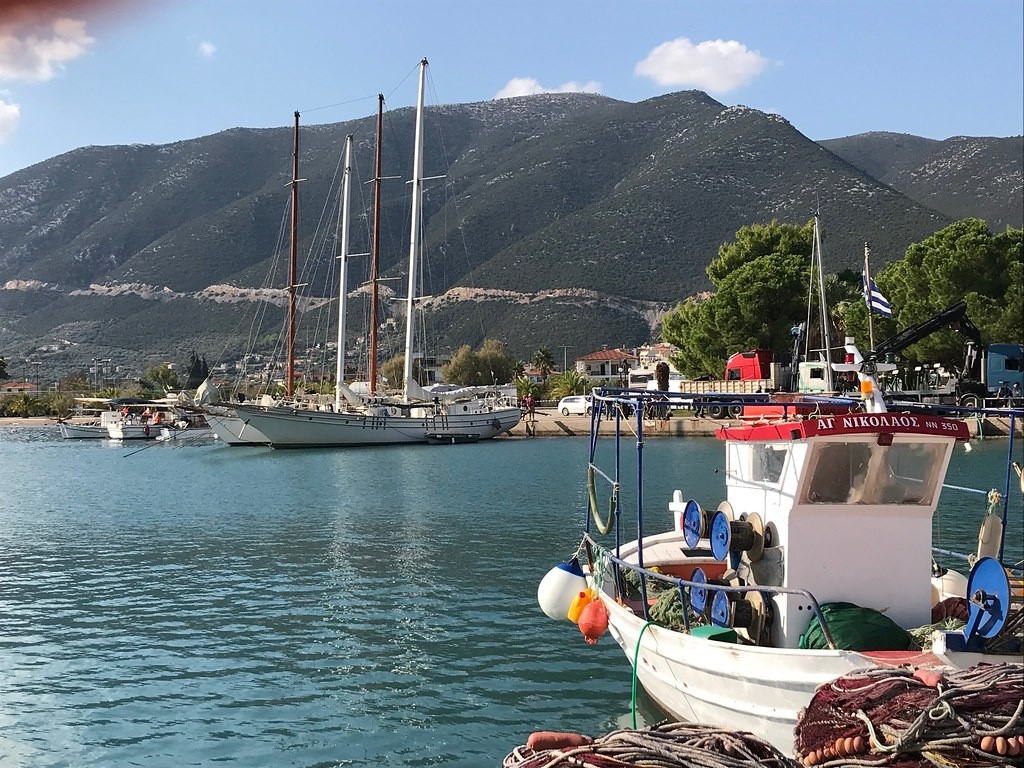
[592,389,670,420]
[520,393,535,421]
[996,381,1023,418]
[504,398,512,407]
[123,406,150,424]
[755,385,761,394]
[329,402,333,413]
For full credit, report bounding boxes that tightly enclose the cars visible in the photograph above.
[558,395,592,416]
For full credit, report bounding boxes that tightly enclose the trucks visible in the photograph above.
[724,348,793,382]
[847,300,1024,418]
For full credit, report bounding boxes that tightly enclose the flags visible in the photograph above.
[864,270,893,314]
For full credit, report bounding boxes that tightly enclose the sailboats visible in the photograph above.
[207,55,524,450]
[738,212,876,424]
[57,91,403,442]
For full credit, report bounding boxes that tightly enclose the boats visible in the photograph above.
[582,388,1024,761]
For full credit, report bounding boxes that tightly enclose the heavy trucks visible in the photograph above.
[680,352,874,420]
